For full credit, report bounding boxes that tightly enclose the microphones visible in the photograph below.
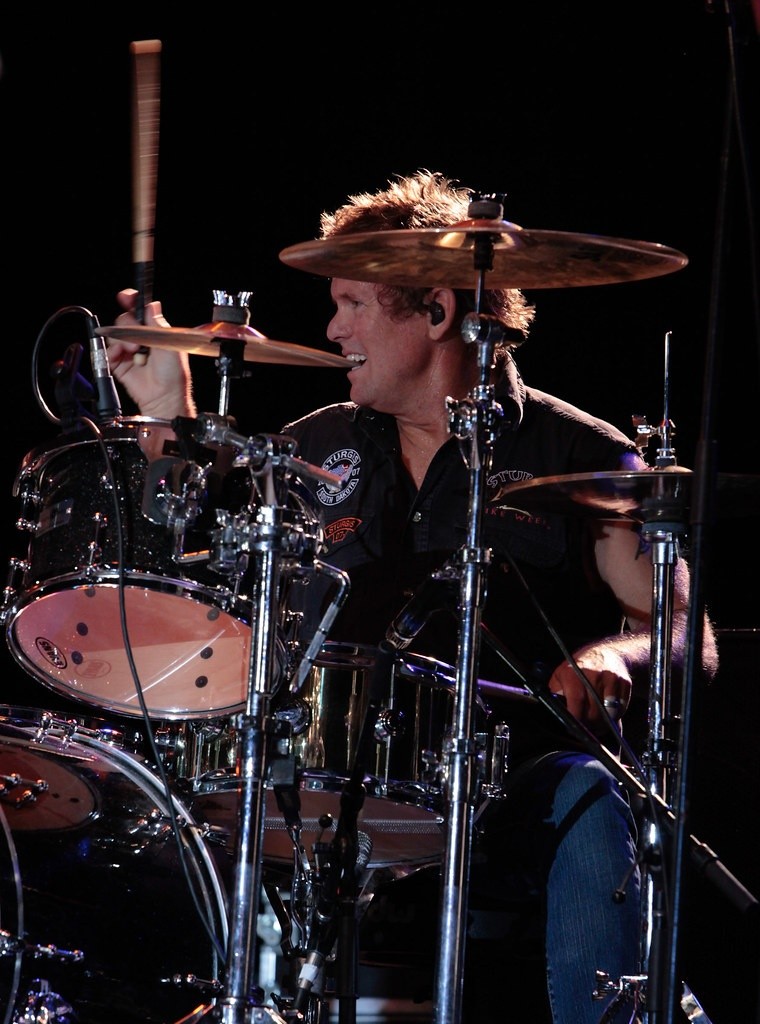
[87,315,122,418]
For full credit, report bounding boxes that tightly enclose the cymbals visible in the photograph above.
[94,325,354,365]
[279,216,688,291]
[496,469,758,520]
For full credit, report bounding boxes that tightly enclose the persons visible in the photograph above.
[99,174,719,1024]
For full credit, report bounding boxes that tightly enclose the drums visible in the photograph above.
[1,705,232,1024]
[188,636,485,868]
[1,416,303,721]
